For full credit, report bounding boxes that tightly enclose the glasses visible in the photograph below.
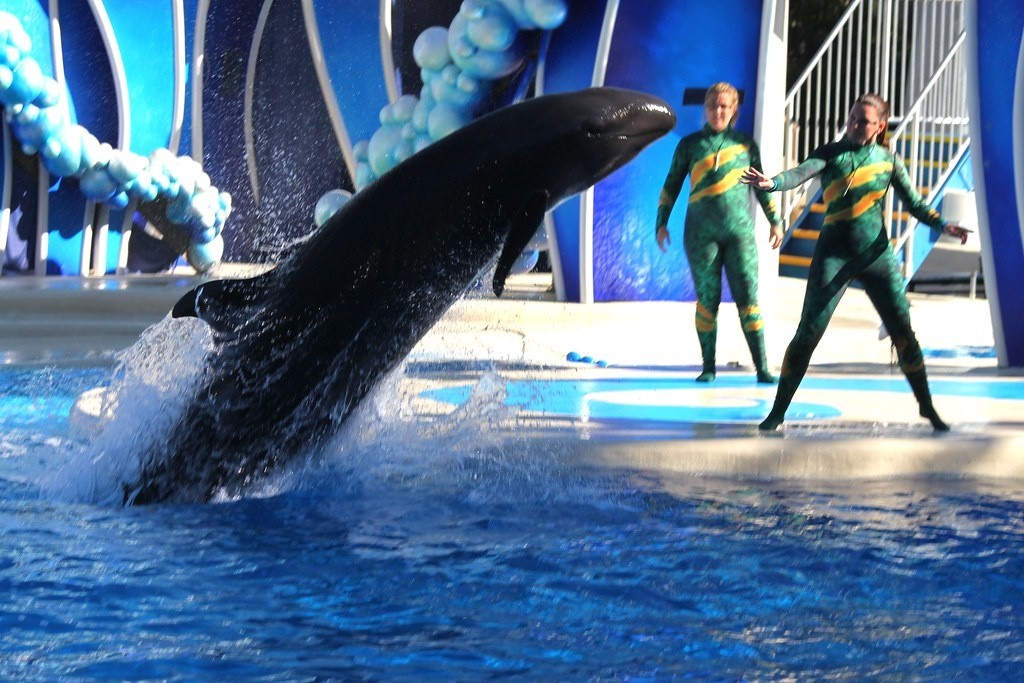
[703,99,738,112]
[848,113,880,127]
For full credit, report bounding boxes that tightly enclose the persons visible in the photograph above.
[657,82,783,384]
[740,94,974,433]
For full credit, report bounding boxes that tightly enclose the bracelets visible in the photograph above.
[769,181,777,191]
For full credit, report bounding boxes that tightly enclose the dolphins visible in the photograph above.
[119,86,676,507]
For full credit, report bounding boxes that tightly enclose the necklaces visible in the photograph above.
[706,133,729,171]
[841,144,875,196]
